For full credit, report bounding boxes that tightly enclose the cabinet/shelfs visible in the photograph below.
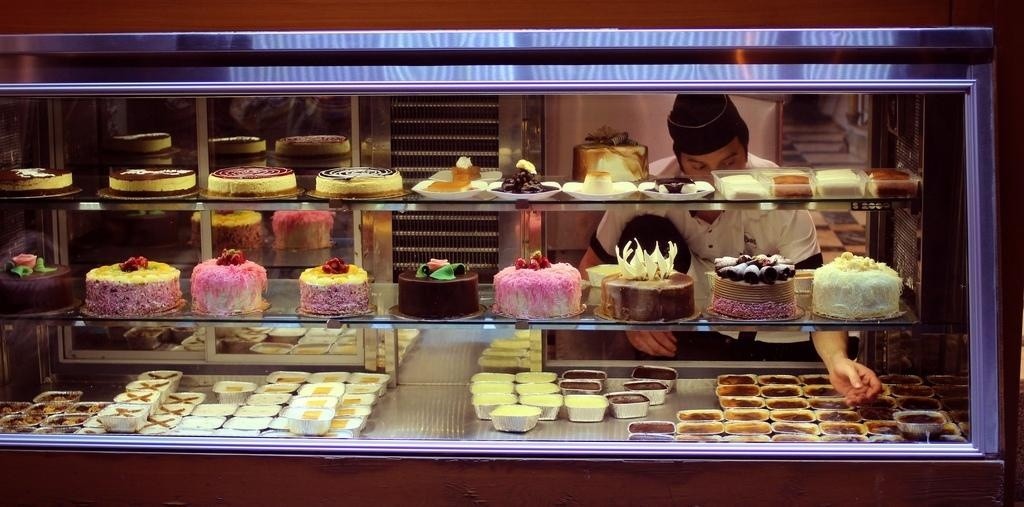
[0,24,1012,506]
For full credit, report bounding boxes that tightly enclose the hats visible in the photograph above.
[666,95,745,158]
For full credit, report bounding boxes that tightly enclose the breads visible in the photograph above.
[720,169,914,199]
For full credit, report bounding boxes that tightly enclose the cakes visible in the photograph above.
[275,135,351,168]
[110,133,174,166]
[191,248,268,314]
[271,211,336,250]
[0,168,74,198]
[811,251,903,320]
[397,258,480,319]
[582,171,613,194]
[191,209,266,249]
[712,254,796,319]
[0,253,73,314]
[492,251,582,317]
[101,207,180,246]
[316,167,403,198]
[208,165,297,197]
[207,136,268,167]
[299,257,369,315]
[108,168,197,197]
[84,255,183,316]
[600,237,696,321]
[425,156,482,194]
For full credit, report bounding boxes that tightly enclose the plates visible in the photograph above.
[560,183,638,200]
[427,167,503,183]
[414,184,489,200]
[488,181,562,200]
[638,181,714,201]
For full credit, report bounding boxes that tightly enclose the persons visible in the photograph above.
[577,94,882,406]
